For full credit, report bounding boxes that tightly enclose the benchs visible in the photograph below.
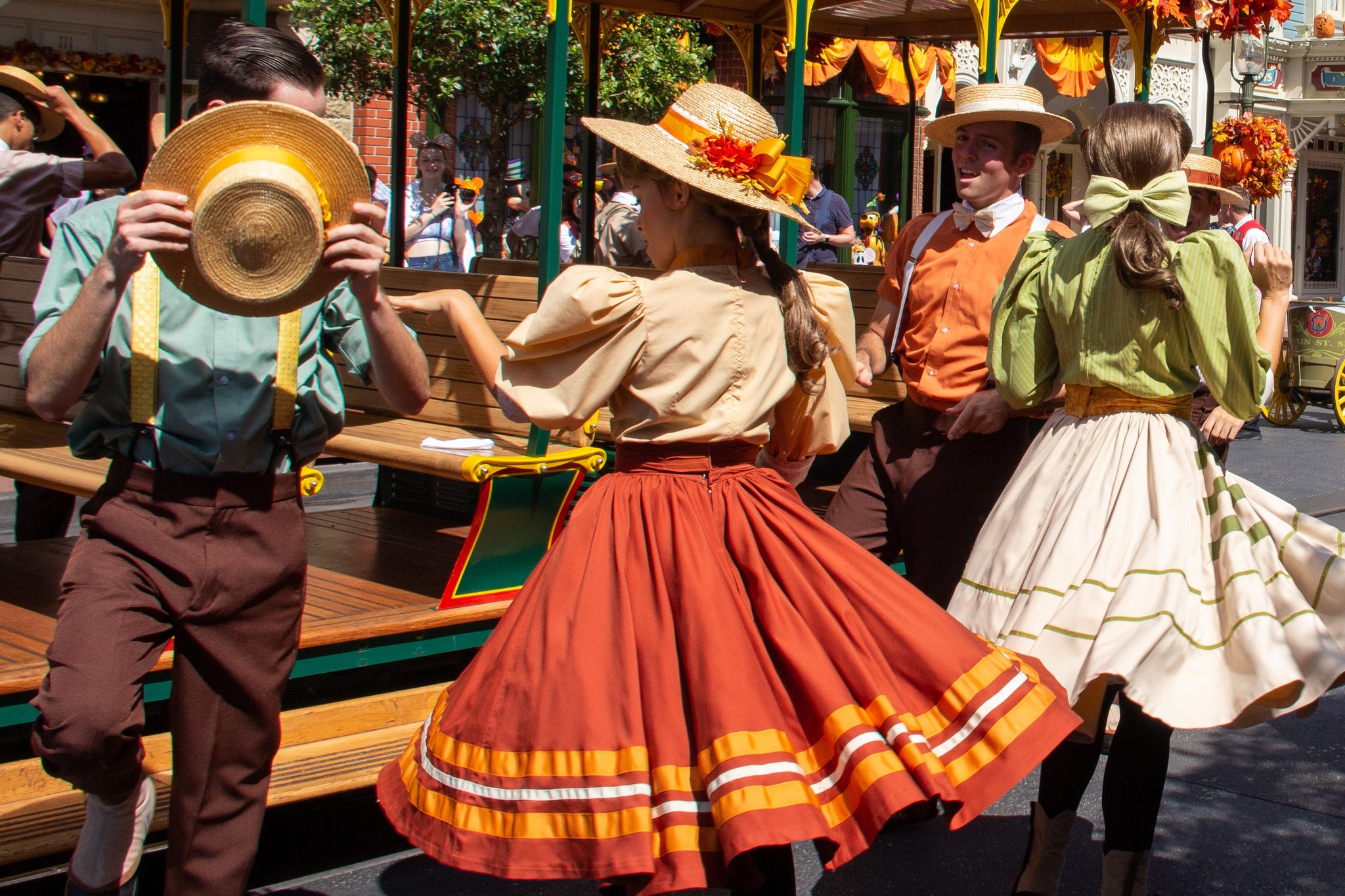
[0,250,114,504]
[303,259,911,612]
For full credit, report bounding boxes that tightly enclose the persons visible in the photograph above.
[507,205,543,261]
[0,63,138,262]
[947,102,1345,896]
[44,138,130,244]
[1220,186,1273,441]
[1209,206,1234,235]
[1061,198,1092,234]
[558,181,612,264]
[405,132,479,274]
[18,20,430,896]
[365,164,378,197]
[1158,153,1247,465]
[375,79,1083,896]
[823,82,1080,831]
[796,154,856,270]
[594,147,656,268]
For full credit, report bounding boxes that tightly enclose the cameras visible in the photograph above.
[444,184,476,208]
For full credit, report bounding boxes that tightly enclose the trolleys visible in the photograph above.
[1261,296,1345,429]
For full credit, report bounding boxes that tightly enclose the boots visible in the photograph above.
[1014,799,1074,896]
[1094,843,1151,895]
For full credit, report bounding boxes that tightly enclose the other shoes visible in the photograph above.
[63,776,156,896]
[1234,416,1262,441]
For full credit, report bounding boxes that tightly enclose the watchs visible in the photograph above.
[824,233,830,244]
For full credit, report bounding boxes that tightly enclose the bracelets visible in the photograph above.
[429,210,437,219]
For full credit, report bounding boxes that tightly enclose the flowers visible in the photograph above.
[677,105,794,200]
[1200,110,1301,208]
[1115,0,1295,45]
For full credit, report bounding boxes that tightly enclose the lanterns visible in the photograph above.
[1314,11,1334,38]
[1218,144,1253,181]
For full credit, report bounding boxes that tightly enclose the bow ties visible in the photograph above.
[952,201,996,238]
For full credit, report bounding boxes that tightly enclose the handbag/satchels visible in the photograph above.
[796,251,812,269]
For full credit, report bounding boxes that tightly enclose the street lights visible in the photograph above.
[1219,24,1275,120]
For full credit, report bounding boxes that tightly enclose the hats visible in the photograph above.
[1179,154,1245,206]
[0,64,64,142]
[925,82,1075,152]
[139,99,373,318]
[582,82,822,237]
[597,148,619,176]
